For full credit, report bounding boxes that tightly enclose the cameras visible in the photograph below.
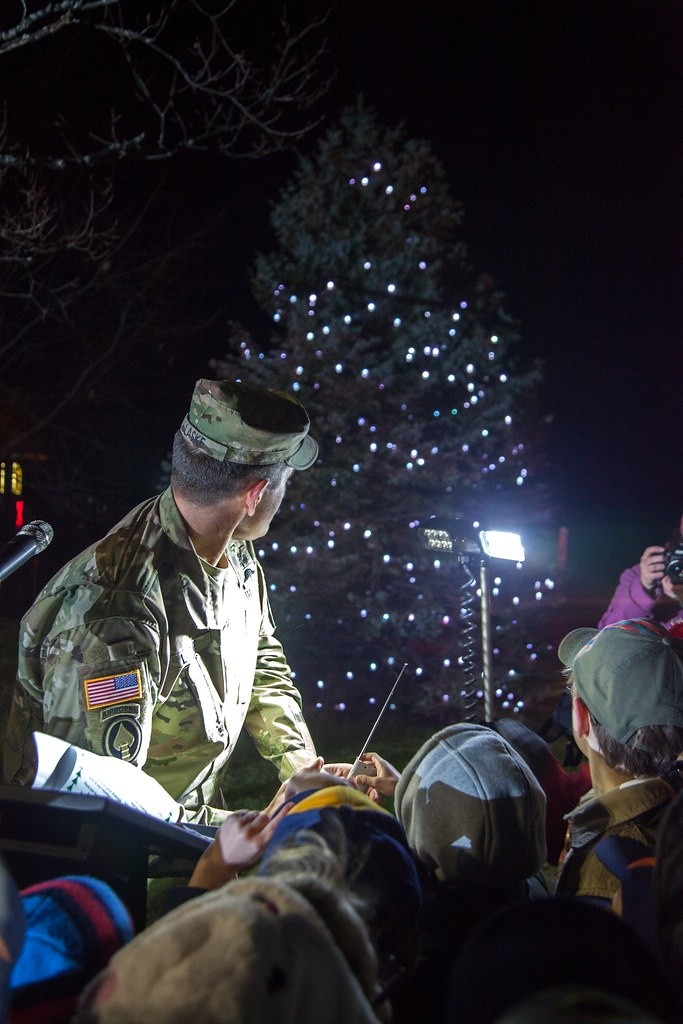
[651,543,683,586]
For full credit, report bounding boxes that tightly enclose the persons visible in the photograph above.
[405,894,680,1023]
[160,787,446,1024]
[485,718,590,865]
[0,379,380,928]
[596,511,683,650]
[263,722,550,1001]
[61,825,402,1024]
[551,618,683,994]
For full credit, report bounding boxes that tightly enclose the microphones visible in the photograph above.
[1,519,54,584]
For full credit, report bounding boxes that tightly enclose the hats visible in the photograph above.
[596,834,659,952]
[258,783,423,946]
[554,615,683,754]
[91,874,378,1024]
[180,378,320,471]
